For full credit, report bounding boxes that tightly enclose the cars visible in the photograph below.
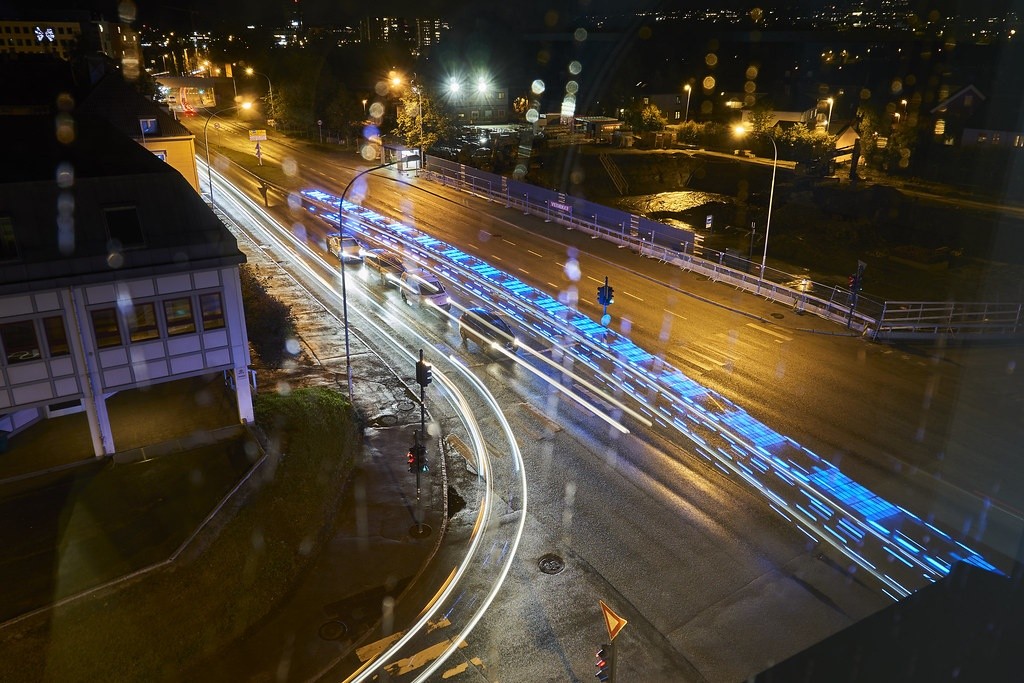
[428,132,492,166]
[399,268,451,312]
[326,233,364,265]
[458,307,519,357]
[168,94,176,103]
[362,249,407,286]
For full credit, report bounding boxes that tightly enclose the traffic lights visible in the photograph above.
[416,362,432,387]
[595,644,612,682]
[606,286,615,305]
[597,286,604,305]
[407,448,417,474]
[418,447,429,473]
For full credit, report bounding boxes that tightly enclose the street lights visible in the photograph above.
[684,84,692,123]
[733,125,778,283]
[246,67,277,131]
[826,97,834,132]
[339,154,422,403]
[203,102,252,210]
[392,77,424,169]
